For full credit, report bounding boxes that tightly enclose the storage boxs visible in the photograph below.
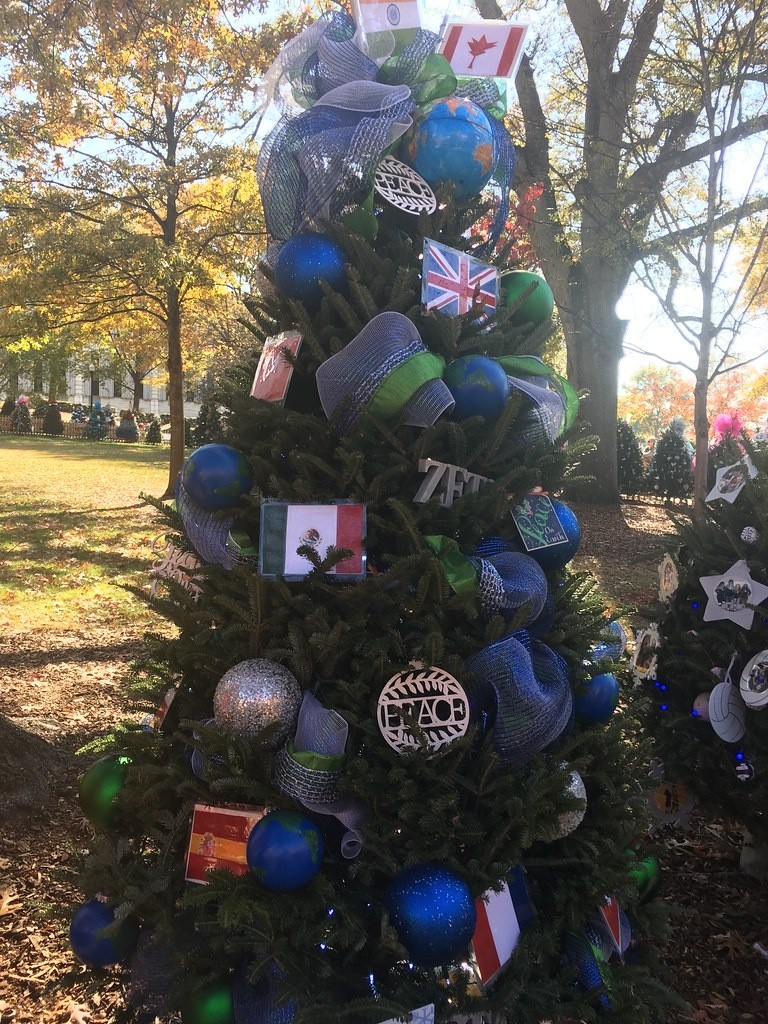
[184,797,272,886]
